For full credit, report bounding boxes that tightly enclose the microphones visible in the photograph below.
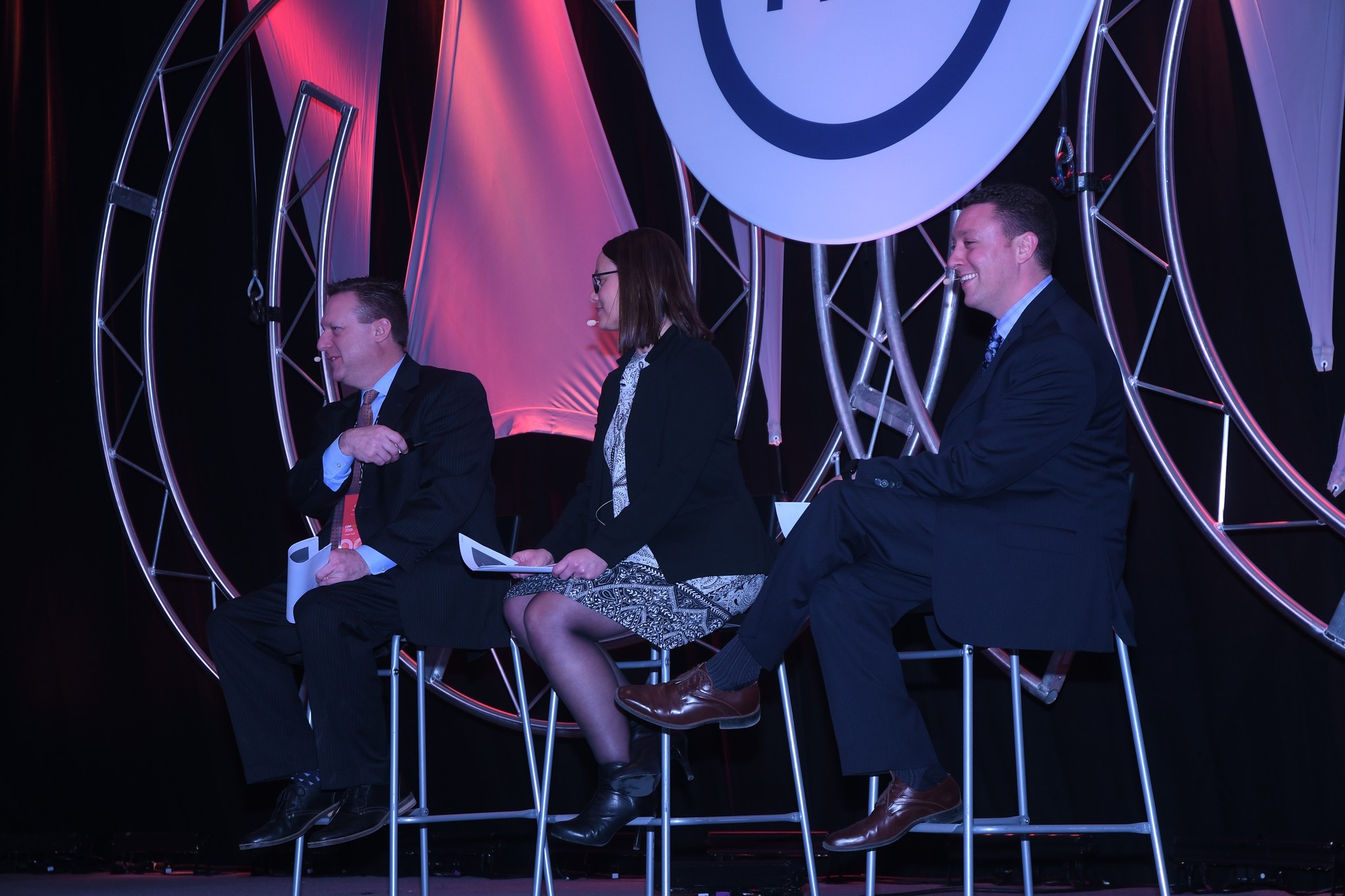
[943,278,959,285]
[587,320,599,326]
[314,356,326,361]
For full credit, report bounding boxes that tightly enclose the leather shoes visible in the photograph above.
[614,662,760,730]
[306,779,416,849]
[822,770,962,851]
[238,774,348,850]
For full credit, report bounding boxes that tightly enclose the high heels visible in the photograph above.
[550,762,659,850]
[609,714,694,798]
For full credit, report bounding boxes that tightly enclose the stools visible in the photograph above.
[292,502,1170,896]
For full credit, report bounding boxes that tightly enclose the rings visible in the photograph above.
[326,576,328,579]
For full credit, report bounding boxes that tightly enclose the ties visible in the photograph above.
[982,323,1004,375]
[329,389,380,551]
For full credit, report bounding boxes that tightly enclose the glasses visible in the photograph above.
[592,270,619,294]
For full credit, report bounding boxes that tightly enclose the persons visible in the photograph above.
[503,228,772,849]
[614,189,1130,850]
[205,276,510,848]
